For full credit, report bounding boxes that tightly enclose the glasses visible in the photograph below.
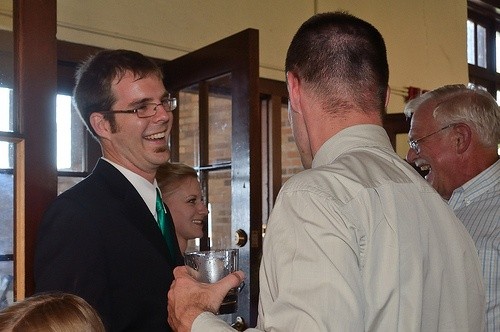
[408,123,455,155]
[100,98,178,118]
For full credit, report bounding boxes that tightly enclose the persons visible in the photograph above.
[404,85,500,332]
[32,51,176,332]
[0,293,106,332]
[155,163,209,255]
[167,13,485,332]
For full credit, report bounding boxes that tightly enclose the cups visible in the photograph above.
[184,249,239,316]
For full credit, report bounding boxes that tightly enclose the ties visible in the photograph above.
[155,188,165,236]
